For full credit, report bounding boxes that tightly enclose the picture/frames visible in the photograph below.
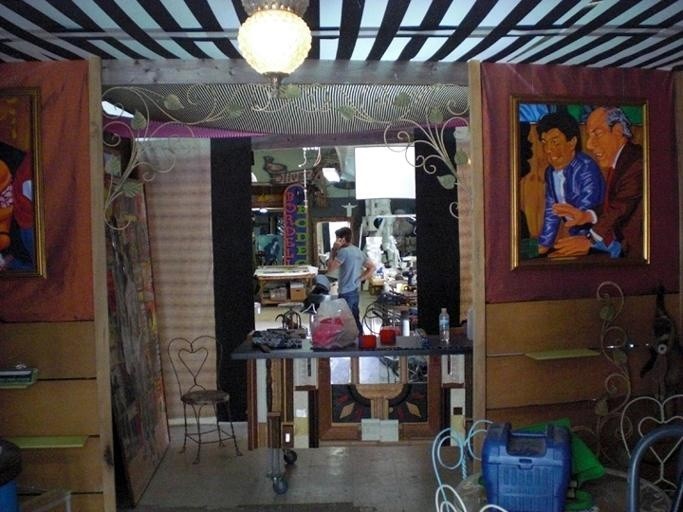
[506,90,656,273]
[0,82,51,283]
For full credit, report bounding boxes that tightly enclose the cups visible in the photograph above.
[358,335,377,348]
[380,329,397,345]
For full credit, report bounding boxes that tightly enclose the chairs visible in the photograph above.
[166,336,242,463]
[433,395,683,510]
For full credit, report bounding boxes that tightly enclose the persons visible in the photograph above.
[323,226,376,336]
[534,110,622,261]
[551,106,642,259]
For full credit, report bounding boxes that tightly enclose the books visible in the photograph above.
[0,365,40,386]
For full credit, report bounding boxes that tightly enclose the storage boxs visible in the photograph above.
[288,281,304,301]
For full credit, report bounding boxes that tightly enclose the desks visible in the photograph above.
[229,331,472,445]
[256,265,317,306]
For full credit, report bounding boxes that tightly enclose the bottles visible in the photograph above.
[413,327,428,344]
[438,308,450,341]
[400,310,409,338]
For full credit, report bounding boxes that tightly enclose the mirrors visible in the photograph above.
[312,215,355,274]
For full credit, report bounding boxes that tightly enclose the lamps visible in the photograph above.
[235,1,314,99]
[322,166,341,183]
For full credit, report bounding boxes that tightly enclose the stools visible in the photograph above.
[277,301,304,329]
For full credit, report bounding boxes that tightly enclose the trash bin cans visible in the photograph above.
[0,437,23,512]
